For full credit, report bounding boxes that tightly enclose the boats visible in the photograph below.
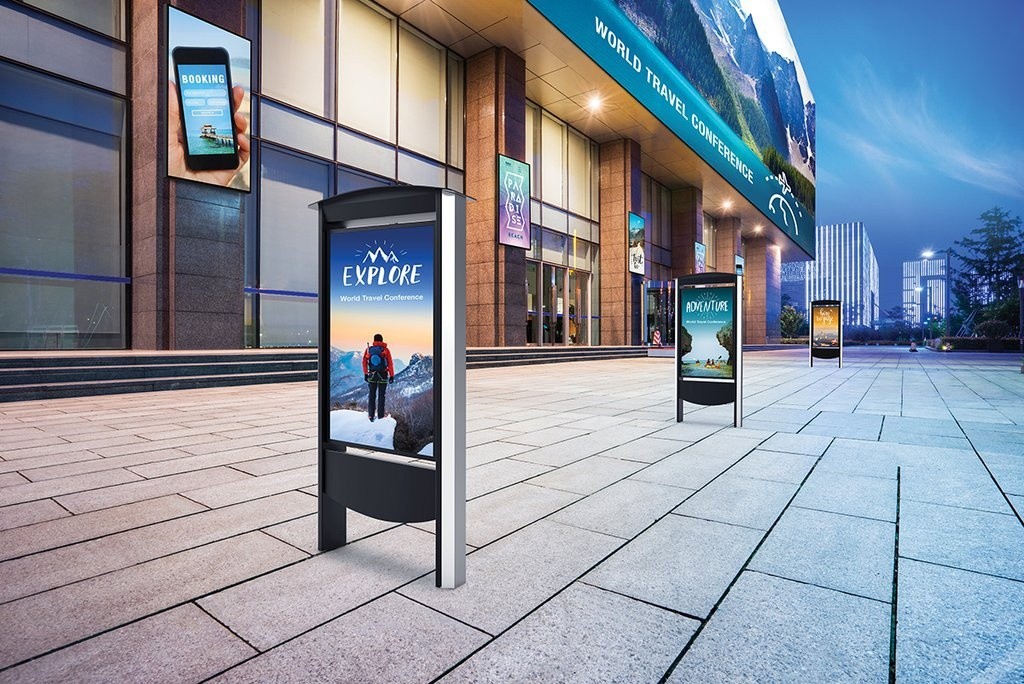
[200,125,216,134]
[696,363,699,366]
[706,364,720,368]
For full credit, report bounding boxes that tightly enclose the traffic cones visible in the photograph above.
[652,326,663,345]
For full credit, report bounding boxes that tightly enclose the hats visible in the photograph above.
[374,334,383,342]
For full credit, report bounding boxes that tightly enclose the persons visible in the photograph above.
[696,356,726,367]
[363,334,394,421]
[168,80,250,187]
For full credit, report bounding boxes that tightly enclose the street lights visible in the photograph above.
[915,287,932,339]
[1017,276,1024,353]
[923,247,950,337]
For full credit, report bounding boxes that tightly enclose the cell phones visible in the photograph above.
[736,264,743,275]
[170,46,239,172]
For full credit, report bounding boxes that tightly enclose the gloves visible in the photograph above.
[389,377,394,384]
[364,374,369,382]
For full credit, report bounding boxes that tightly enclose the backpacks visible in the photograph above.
[368,346,388,372]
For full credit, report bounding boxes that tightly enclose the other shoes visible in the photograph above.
[368,415,375,419]
[378,413,388,419]
